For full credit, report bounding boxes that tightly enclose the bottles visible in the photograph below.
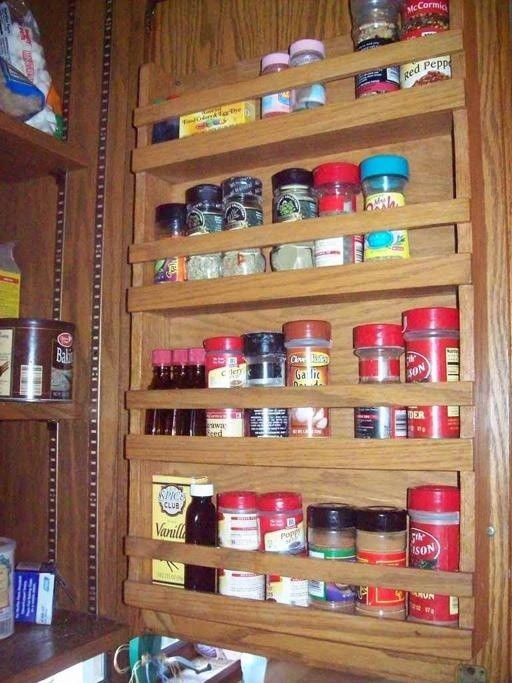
[144,348,207,437]
[351,321,408,438]
[260,51,294,118]
[288,38,328,111]
[311,160,362,268]
[359,153,412,261]
[184,482,218,594]
[348,0,401,98]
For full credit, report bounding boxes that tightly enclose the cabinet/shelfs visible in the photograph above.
[118,0,512,683]
[0,0,94,682]
